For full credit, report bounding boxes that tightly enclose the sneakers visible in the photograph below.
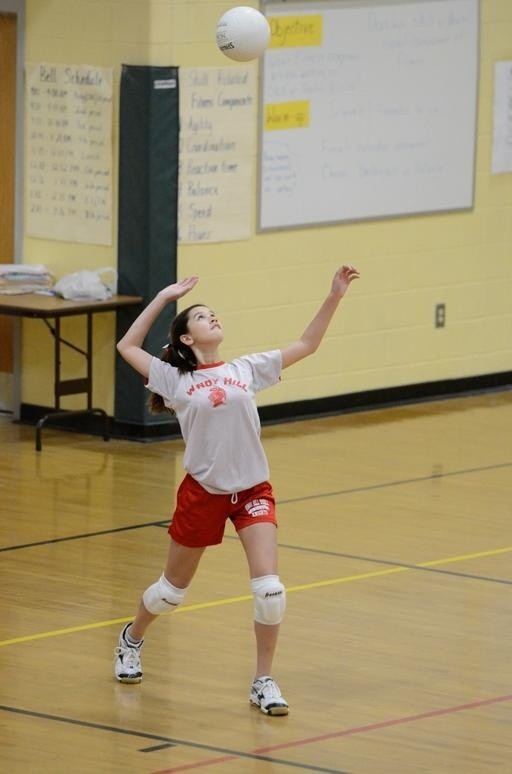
[248,675,289,716]
[114,619,145,684]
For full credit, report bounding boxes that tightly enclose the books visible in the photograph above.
[0,262,53,297]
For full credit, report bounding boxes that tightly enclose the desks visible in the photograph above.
[1,281,143,452]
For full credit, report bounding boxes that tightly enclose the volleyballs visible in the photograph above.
[216,5,272,60]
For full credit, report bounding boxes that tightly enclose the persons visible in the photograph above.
[115,263,360,714]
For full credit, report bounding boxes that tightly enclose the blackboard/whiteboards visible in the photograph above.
[258,0,481,234]
